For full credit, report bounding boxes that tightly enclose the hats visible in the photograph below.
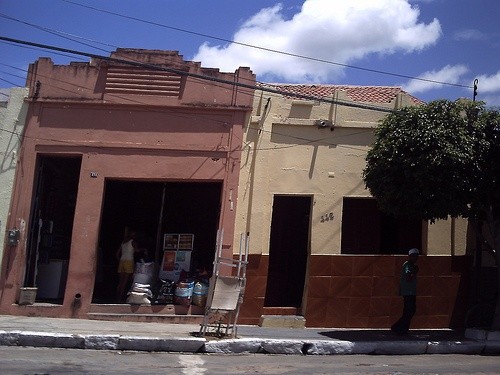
[408,248,420,255]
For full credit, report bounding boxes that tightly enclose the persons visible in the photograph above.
[390,248,420,336]
[114,229,138,304]
